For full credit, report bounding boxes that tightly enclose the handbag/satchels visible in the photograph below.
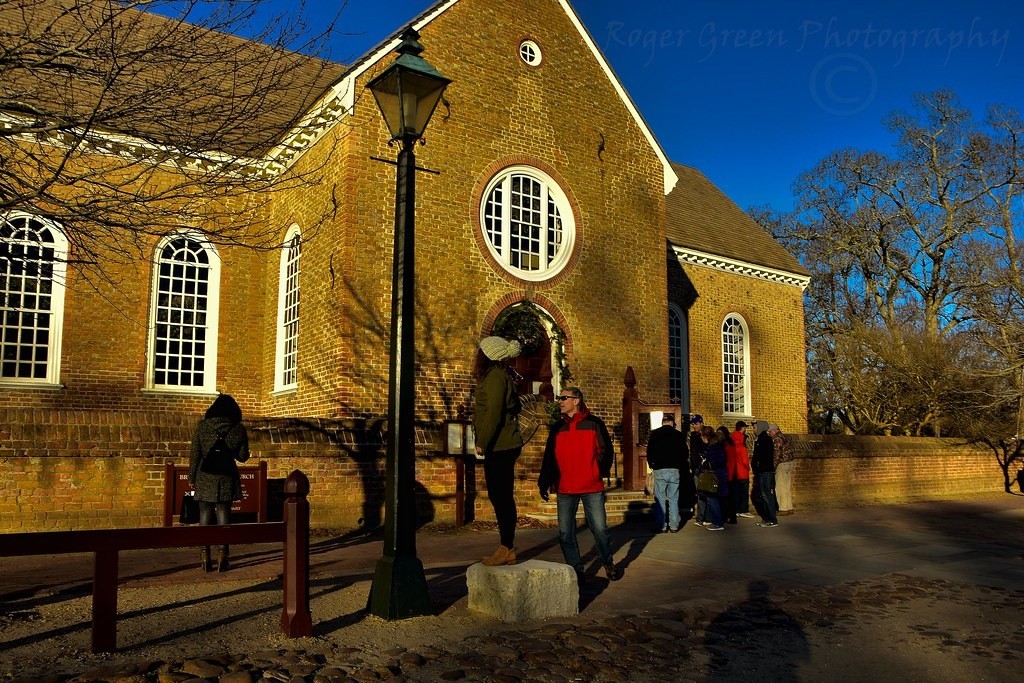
[697,470,718,493]
[179,491,200,524]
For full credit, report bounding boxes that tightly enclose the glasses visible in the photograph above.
[556,395,577,401]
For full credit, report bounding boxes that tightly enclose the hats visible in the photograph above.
[662,416,676,428]
[690,414,702,423]
[736,421,749,427]
[751,422,757,426]
[768,423,779,432]
[480,337,522,362]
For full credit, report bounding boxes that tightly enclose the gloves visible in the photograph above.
[539,486,550,501]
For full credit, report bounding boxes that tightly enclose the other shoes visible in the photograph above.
[740,512,755,518]
[762,522,778,527]
[671,530,676,533]
[706,524,724,531]
[702,522,712,526]
[736,513,740,516]
[776,511,788,516]
[694,522,703,527]
[755,521,767,526]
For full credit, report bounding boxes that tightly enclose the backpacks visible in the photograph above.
[201,423,235,475]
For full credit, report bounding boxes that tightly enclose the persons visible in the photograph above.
[731,420,756,518]
[690,414,737,531]
[538,387,617,581]
[750,421,779,528]
[471,336,522,567]
[769,424,794,516]
[188,394,250,572]
[646,415,685,533]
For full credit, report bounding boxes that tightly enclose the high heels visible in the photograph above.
[217,550,230,573]
[199,550,211,573]
[483,545,516,566]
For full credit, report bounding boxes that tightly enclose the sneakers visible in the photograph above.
[603,563,617,581]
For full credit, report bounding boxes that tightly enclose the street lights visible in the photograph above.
[364,26,458,621]
